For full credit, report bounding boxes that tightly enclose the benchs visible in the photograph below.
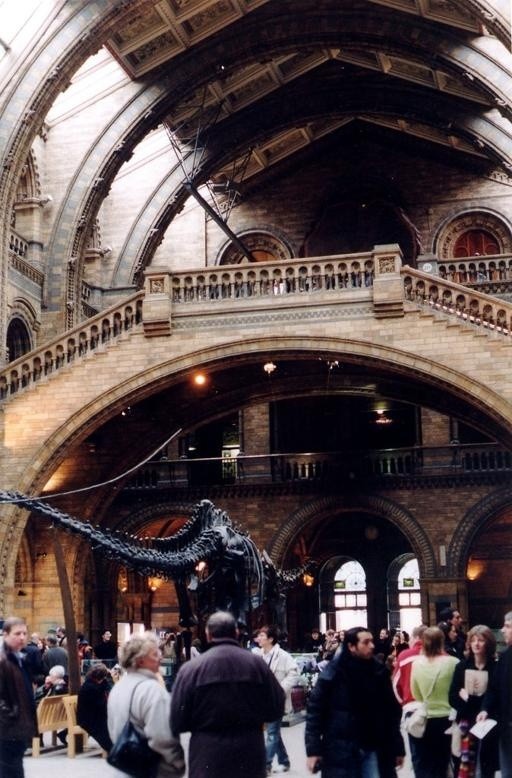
[28,694,109,759]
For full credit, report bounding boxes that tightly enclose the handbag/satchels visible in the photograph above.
[106,718,163,778]
[404,706,429,738]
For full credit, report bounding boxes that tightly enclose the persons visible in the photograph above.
[171,608,285,778]
[105,629,184,777]
[25,625,203,702]
[257,624,299,776]
[305,608,512,777]
[75,663,112,752]
[0,616,39,777]
